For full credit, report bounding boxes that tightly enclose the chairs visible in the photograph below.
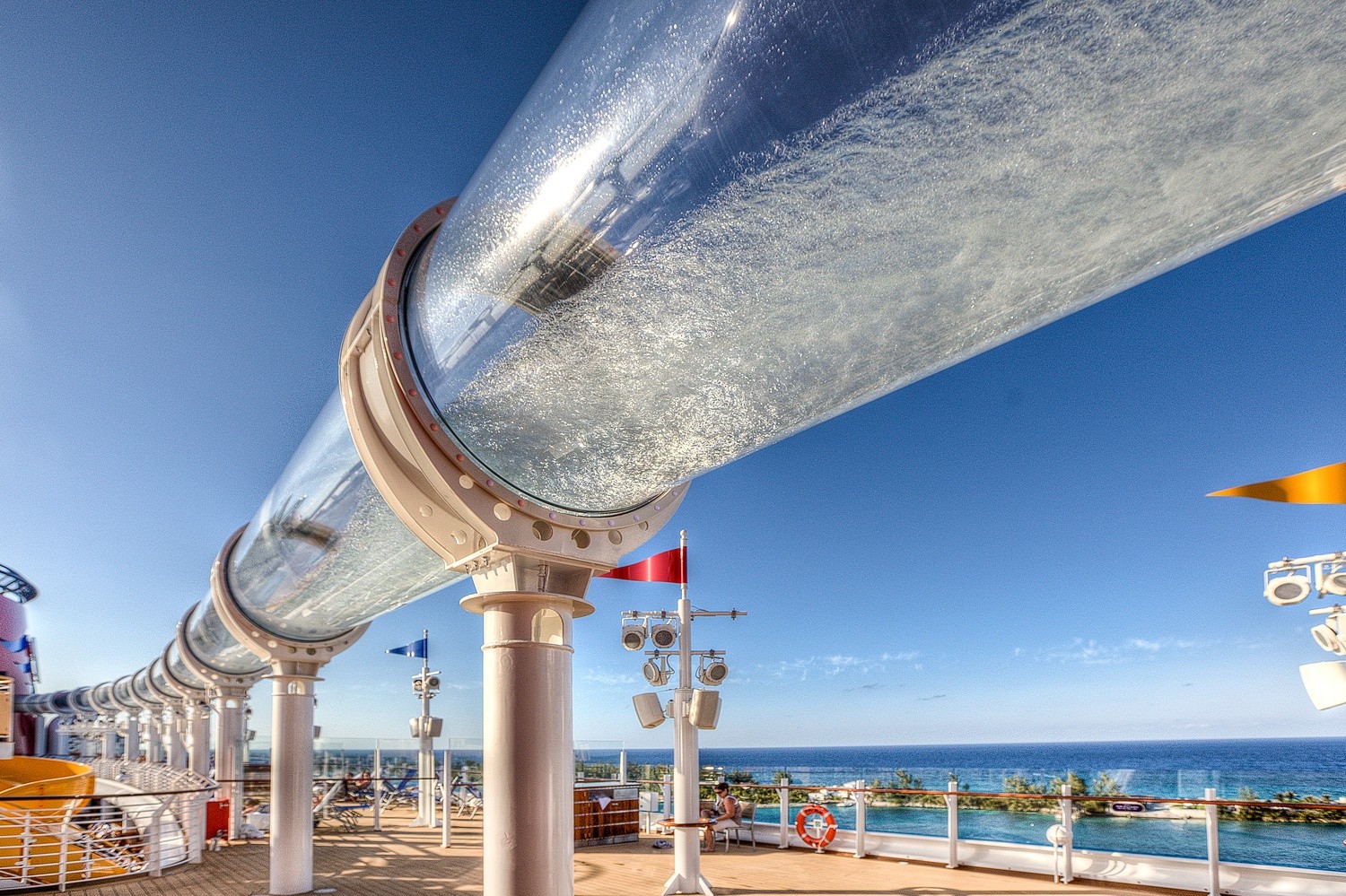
[712,801,756,853]
[314,769,483,834]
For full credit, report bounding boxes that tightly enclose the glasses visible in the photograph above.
[715,791,723,794]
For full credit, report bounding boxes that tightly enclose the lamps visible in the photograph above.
[696,654,727,686]
[650,611,677,649]
[621,611,648,651]
[1264,557,1311,606]
[1309,606,1346,656]
[1315,552,1346,598]
[412,676,440,699]
[642,651,674,686]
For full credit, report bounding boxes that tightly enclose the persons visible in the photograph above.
[88,818,139,855]
[242,799,259,818]
[701,783,741,853]
[341,771,358,802]
[356,772,371,785]
